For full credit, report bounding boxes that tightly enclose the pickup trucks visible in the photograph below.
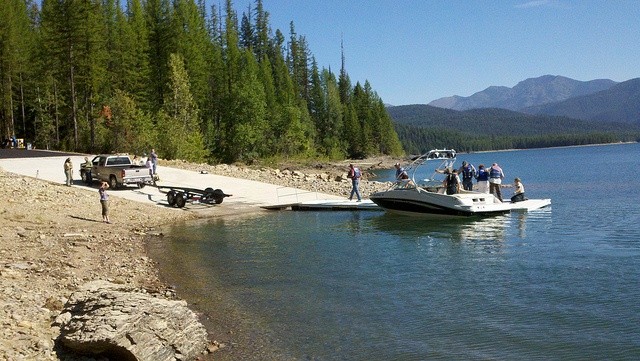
[79,155,152,190]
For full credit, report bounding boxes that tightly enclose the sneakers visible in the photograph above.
[356,201,361,202]
[107,222,112,224]
[103,221,107,223]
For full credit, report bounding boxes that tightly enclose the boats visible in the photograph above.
[368,148,552,217]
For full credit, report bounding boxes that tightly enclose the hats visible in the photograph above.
[348,164,354,167]
[394,163,400,166]
[514,176,520,182]
[462,161,467,166]
[452,169,459,172]
[151,149,155,152]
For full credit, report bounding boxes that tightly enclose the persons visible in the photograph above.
[99,182,112,224]
[139,153,147,165]
[64,157,73,187]
[394,162,404,189]
[146,157,154,182]
[475,164,489,194]
[10,136,17,148]
[133,155,138,165]
[442,169,460,195]
[508,176,528,202]
[84,157,93,187]
[486,163,504,203]
[402,175,414,189]
[347,163,362,202]
[458,161,476,192]
[150,149,158,175]
